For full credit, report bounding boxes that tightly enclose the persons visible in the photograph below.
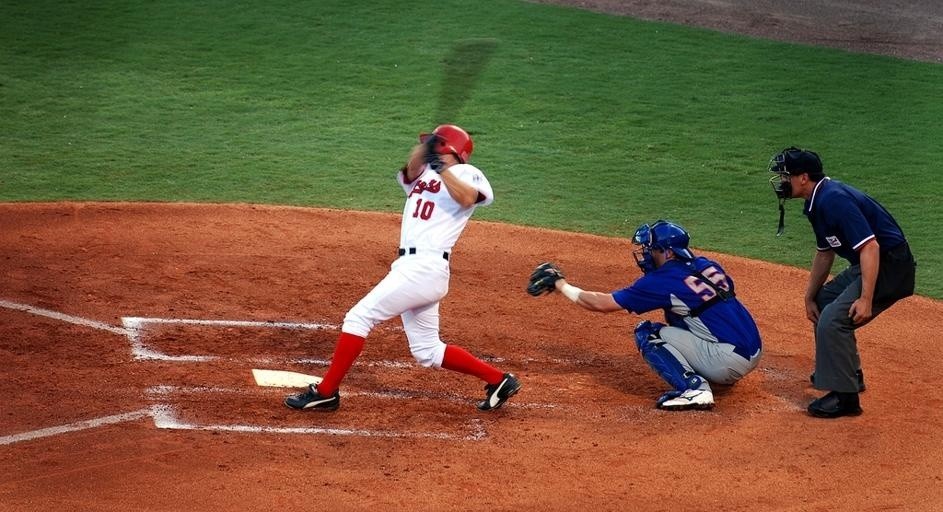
[284,124,522,410]
[768,146,915,418]
[527,219,763,410]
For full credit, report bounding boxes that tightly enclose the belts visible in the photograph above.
[398,248,449,260]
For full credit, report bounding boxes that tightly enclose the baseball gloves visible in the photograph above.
[527,264,562,296]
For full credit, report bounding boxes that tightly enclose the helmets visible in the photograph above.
[420,125,473,164]
[767,146,823,237]
[632,220,695,275]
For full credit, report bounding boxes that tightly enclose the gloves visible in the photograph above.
[425,136,446,173]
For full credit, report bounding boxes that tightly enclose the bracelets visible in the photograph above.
[561,284,584,303]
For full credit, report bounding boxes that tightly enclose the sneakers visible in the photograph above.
[661,387,715,410]
[476,372,521,412]
[811,369,865,392]
[284,383,339,411]
[807,391,860,417]
